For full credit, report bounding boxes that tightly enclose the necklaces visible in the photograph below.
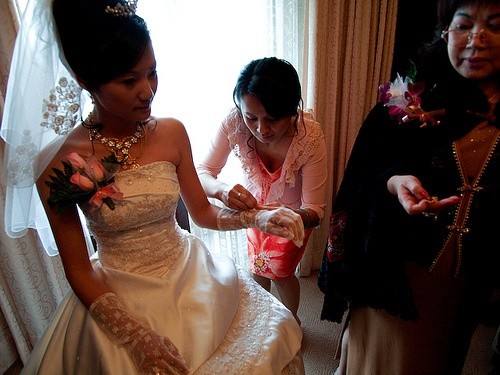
[84,113,154,169]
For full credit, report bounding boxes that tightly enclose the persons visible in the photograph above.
[316,0,499,374]
[21,0,310,375]
[191,56,328,331]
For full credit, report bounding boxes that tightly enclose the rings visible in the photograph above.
[237,192,242,200]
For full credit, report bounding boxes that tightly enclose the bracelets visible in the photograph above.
[297,207,311,229]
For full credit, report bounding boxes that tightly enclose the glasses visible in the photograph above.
[439,23,500,49]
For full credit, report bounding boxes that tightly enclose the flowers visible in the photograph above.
[378,59,445,127]
[43,152,123,216]
